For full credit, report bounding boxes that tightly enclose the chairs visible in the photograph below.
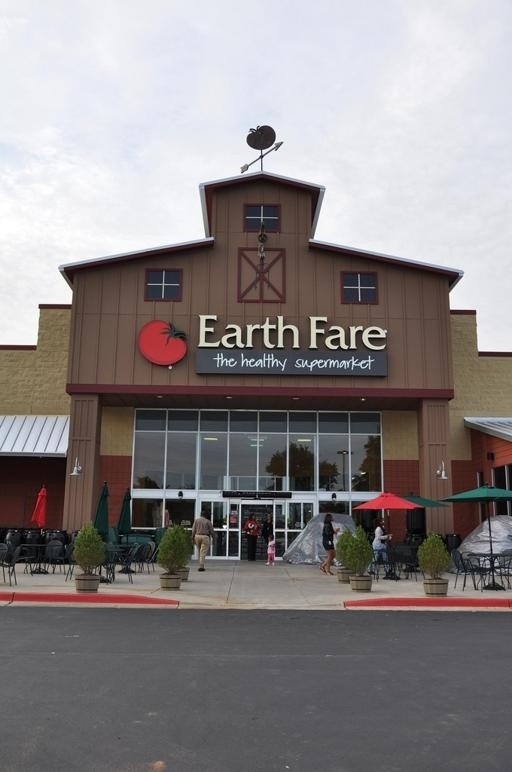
[372,542,512,592]
[0,528,159,587]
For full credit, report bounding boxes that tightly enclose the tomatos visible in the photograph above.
[139,319,189,366]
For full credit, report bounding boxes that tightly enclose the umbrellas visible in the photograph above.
[115,485,131,567]
[398,491,450,529]
[93,480,109,574]
[352,493,424,527]
[439,482,511,589]
[31,484,48,569]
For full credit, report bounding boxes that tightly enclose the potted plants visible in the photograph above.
[73,520,105,593]
[158,525,185,590]
[346,525,373,592]
[416,532,450,595]
[335,527,356,583]
[174,527,194,579]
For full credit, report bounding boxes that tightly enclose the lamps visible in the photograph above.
[435,459,449,480]
[68,457,81,476]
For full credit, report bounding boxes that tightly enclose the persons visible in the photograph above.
[318,513,340,575]
[260,512,273,545]
[264,534,276,566]
[366,516,396,578]
[191,510,216,571]
[242,513,259,561]
